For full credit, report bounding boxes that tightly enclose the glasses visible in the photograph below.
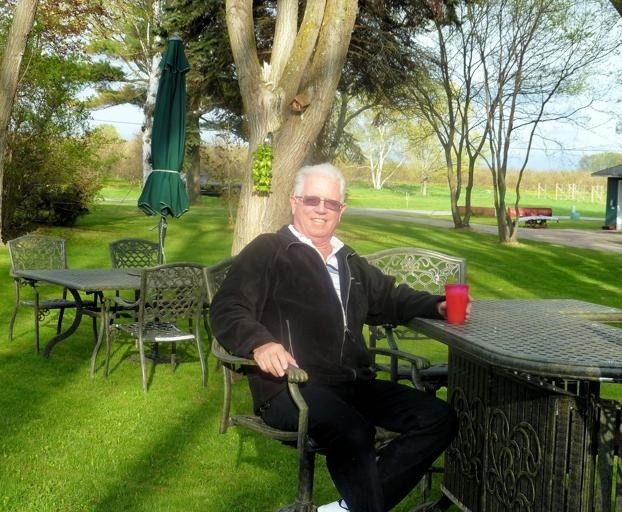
[295,195,343,212]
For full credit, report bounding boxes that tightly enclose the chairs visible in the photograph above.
[354,247,464,482]
[7,231,209,394]
[203,256,430,512]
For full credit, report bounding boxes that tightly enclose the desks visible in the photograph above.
[406,297,621,512]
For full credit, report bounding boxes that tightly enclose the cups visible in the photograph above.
[443,283,470,325]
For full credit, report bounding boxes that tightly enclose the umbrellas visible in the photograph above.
[137,32,191,264]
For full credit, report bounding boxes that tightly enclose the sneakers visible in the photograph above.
[317,499,351,512]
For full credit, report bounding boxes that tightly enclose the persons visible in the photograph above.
[209,162,473,512]
